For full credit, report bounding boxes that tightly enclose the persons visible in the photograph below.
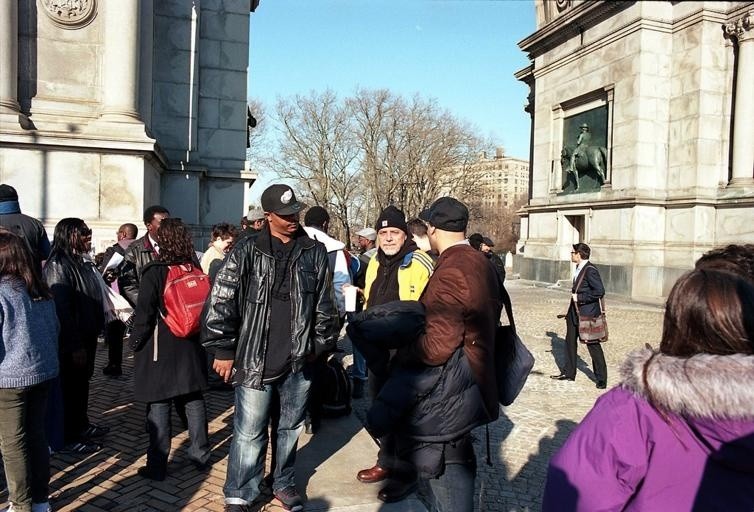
[548,242,608,388]
[566,121,591,175]
[539,237,753,512]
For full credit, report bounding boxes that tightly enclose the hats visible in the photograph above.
[418,196,470,232]
[481,236,495,247]
[260,183,308,217]
[374,205,408,234]
[354,227,378,241]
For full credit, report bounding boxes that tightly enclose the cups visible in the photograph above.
[344,286,357,313]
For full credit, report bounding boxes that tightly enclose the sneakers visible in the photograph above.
[138,464,167,481]
[59,422,110,454]
[196,463,213,474]
[5,502,53,512]
[273,487,305,512]
[224,503,249,512]
[351,376,366,400]
[101,364,123,377]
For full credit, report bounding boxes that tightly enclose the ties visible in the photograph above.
[574,268,581,282]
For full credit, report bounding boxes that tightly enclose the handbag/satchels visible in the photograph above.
[496,323,536,407]
[578,314,609,344]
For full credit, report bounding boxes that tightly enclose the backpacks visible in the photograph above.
[158,261,212,338]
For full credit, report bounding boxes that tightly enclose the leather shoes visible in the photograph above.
[595,383,607,390]
[550,375,575,382]
[356,463,390,484]
[377,479,418,504]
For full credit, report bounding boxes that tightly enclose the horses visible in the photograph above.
[561,144,607,193]
